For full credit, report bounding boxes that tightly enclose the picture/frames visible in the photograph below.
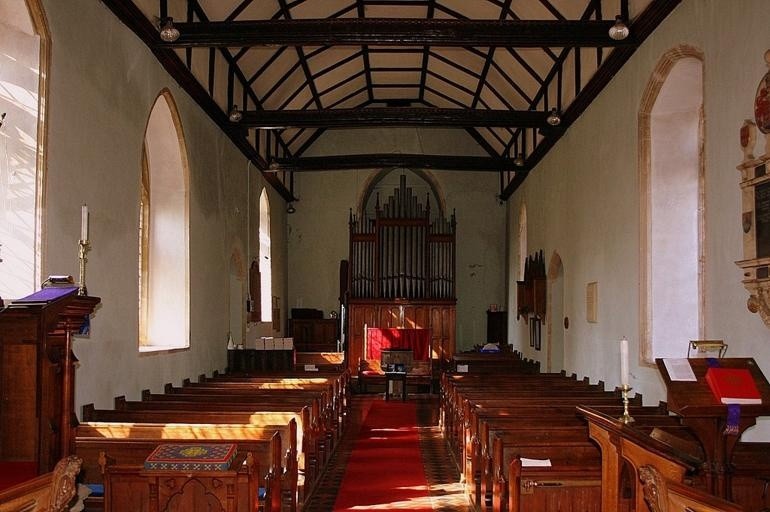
[530,317,541,351]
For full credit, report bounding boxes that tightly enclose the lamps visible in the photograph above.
[269,156,279,170]
[609,15,632,41]
[546,107,563,126]
[514,153,527,167]
[151,14,180,43]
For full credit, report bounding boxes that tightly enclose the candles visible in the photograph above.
[81,203,89,240]
[620,335,630,386]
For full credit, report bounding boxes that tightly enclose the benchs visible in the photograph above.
[357,356,434,394]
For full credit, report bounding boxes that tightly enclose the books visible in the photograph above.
[663,358,698,382]
[705,367,763,404]
[11,287,79,303]
[385,362,396,374]
[256,336,294,350]
[395,363,405,374]
[7,302,49,309]
